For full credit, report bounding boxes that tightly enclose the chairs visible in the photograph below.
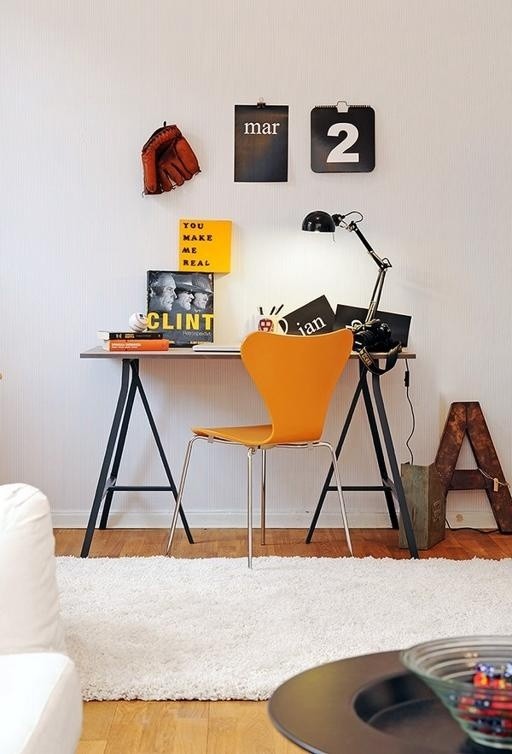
[0,483,83,754]
[166,328,353,570]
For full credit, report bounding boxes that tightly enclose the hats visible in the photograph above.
[171,273,213,295]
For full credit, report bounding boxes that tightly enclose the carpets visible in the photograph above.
[55,557,512,700]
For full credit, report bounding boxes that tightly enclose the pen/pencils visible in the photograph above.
[259,305,284,315]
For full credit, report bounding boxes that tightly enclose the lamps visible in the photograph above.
[301,211,392,353]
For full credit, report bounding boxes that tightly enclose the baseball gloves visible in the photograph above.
[142,121,202,194]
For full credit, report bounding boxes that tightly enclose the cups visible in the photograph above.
[256,315,289,335]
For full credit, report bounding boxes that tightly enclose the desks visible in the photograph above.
[81,338,419,558]
[265,648,510,753]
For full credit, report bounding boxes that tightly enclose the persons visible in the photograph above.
[174,274,195,310]
[148,271,176,312]
[193,273,213,310]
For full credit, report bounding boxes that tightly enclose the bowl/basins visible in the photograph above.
[398,634,512,750]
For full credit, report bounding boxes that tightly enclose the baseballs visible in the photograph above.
[129,312,149,331]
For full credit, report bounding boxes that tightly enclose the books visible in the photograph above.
[102,340,170,353]
[97,331,164,341]
[144,270,214,344]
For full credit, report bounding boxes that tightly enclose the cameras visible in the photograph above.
[352,318,393,352]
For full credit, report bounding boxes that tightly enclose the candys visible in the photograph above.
[449,662,512,745]
